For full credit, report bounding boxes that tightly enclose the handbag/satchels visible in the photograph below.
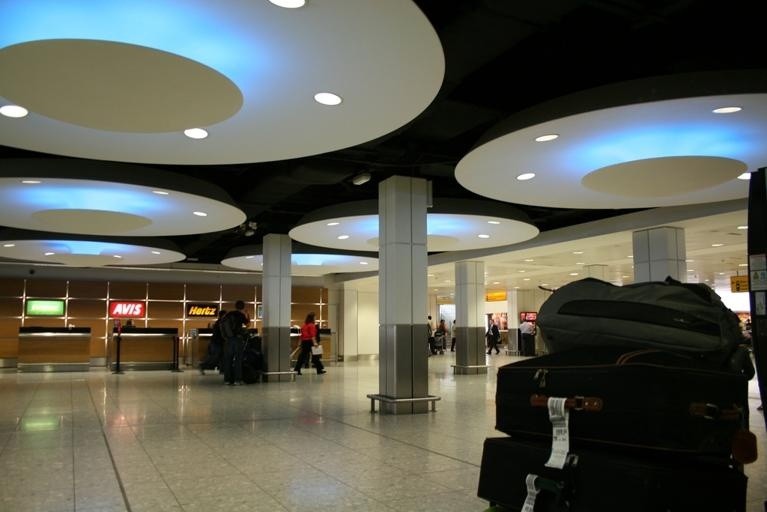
[218,316,235,340]
[289,346,304,360]
[536,276,745,358]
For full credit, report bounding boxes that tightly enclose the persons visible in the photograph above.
[197,310,228,376]
[485,319,500,355]
[291,311,326,376]
[222,300,250,386]
[519,318,536,356]
[427,315,456,355]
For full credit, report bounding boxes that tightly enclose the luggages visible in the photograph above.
[477,436,749,512]
[495,355,758,465]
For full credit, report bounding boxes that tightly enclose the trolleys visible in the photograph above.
[433,330,446,355]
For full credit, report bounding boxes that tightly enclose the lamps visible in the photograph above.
[0,159,247,238]
[454,62,767,217]
[289,198,540,252]
[0,0,446,165]
[0,238,187,268]
[220,243,379,277]
